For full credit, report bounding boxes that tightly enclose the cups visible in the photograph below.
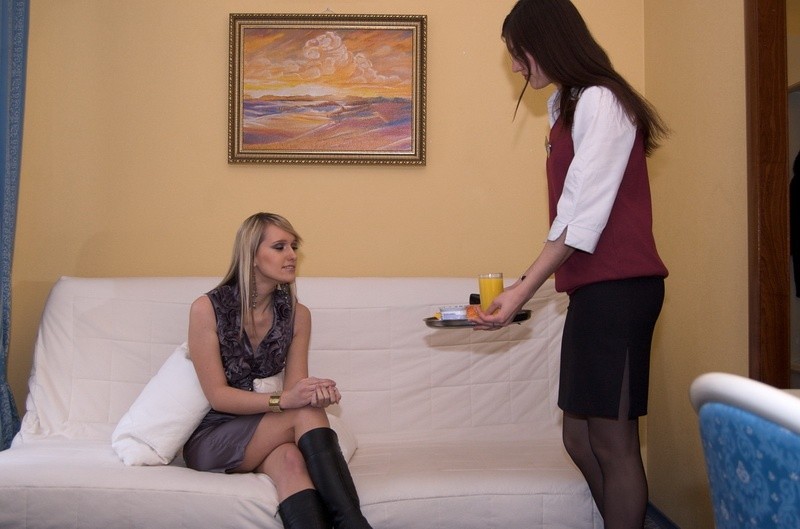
[478,271,504,315]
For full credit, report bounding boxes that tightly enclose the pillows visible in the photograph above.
[252,363,358,467]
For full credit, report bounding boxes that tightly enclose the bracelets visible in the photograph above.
[521,274,526,281]
[268,391,285,414]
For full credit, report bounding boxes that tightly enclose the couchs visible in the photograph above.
[689,371,800,529]
[0,275,605,529]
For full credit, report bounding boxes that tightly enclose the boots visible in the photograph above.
[274,427,374,529]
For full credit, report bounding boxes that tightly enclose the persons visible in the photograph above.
[178,212,376,529]
[465,0,679,528]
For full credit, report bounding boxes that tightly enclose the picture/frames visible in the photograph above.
[227,11,428,168]
